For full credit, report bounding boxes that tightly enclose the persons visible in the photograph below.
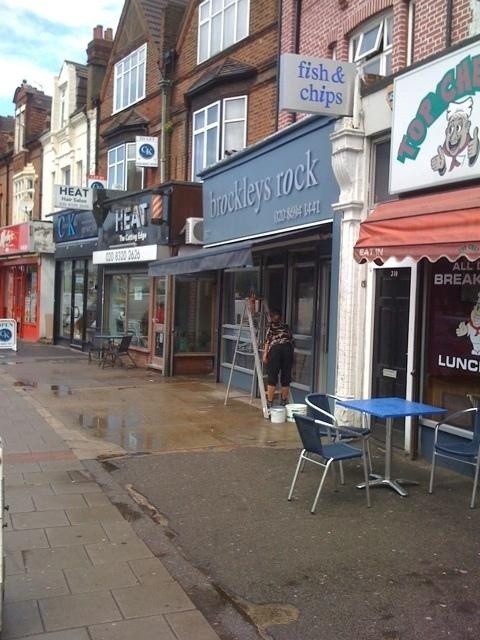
[259,309,295,409]
[156,302,164,356]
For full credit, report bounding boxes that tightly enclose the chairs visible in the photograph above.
[305,393,372,485]
[114,336,138,367]
[428,407,480,508]
[288,414,371,513]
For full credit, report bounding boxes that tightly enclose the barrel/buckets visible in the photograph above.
[266,405,286,424]
[286,403,308,422]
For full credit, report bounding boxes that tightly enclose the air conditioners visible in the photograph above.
[185,217,205,246]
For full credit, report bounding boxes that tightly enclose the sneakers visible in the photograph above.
[280,399,286,406]
[267,401,272,408]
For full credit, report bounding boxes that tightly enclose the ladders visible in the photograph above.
[224,296,295,419]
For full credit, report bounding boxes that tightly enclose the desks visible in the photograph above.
[336,397,448,497]
[96,336,125,370]
